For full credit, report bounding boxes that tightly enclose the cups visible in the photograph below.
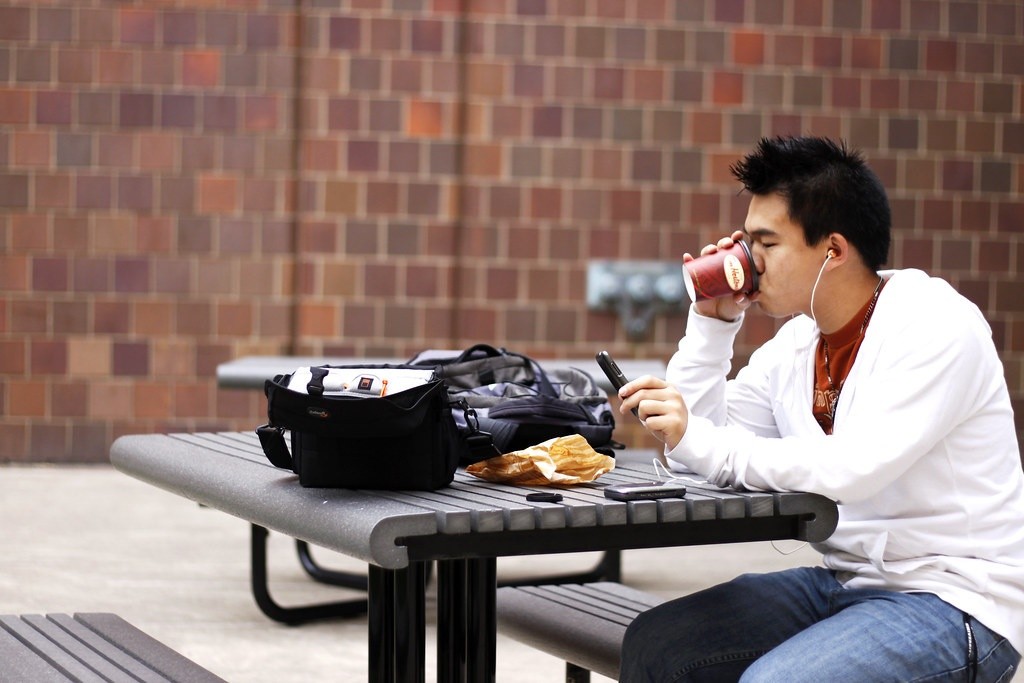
[682,239,758,303]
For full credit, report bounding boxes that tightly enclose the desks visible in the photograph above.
[217,355,665,628]
[110,429,839,683]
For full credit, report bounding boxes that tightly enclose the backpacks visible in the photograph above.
[406,344,626,459]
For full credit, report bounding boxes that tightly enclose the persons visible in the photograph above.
[618,135,1024,683]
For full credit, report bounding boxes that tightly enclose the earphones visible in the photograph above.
[828,249,836,259]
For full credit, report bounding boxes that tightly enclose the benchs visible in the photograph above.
[496,581,667,683]
[0,612,229,683]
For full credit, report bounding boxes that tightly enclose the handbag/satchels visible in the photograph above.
[252,364,499,493]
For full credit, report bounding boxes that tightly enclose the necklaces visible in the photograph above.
[824,275,882,428]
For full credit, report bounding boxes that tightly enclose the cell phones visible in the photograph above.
[603,482,687,502]
[596,350,659,421]
